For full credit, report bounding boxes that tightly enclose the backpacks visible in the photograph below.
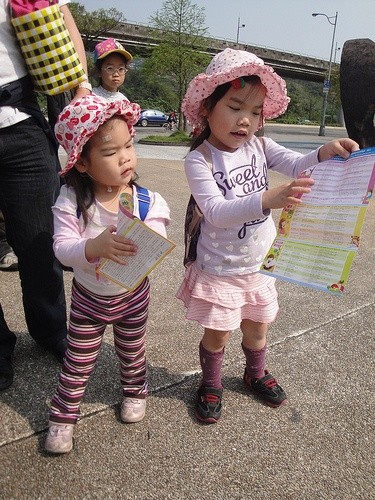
[183,137,266,270]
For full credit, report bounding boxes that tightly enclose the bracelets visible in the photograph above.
[73,82,92,93]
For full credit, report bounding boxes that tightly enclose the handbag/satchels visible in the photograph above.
[10,0,88,95]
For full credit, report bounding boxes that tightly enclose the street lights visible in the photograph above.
[312,11,339,137]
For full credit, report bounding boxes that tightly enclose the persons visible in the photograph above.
[164,109,175,130]
[0,0,133,391]
[175,48,360,423]
[44,95,170,453]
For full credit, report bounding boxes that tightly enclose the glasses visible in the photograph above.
[101,65,128,74]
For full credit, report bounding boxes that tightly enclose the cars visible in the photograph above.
[133,109,174,127]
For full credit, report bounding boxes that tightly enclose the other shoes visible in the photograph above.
[0,251,19,272]
[44,338,68,363]
[0,332,16,391]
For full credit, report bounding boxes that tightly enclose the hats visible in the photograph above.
[182,48,290,131]
[93,38,133,64]
[53,95,141,176]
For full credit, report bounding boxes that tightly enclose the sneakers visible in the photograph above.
[195,379,224,423]
[121,397,146,422]
[45,420,74,453]
[243,367,287,407]
